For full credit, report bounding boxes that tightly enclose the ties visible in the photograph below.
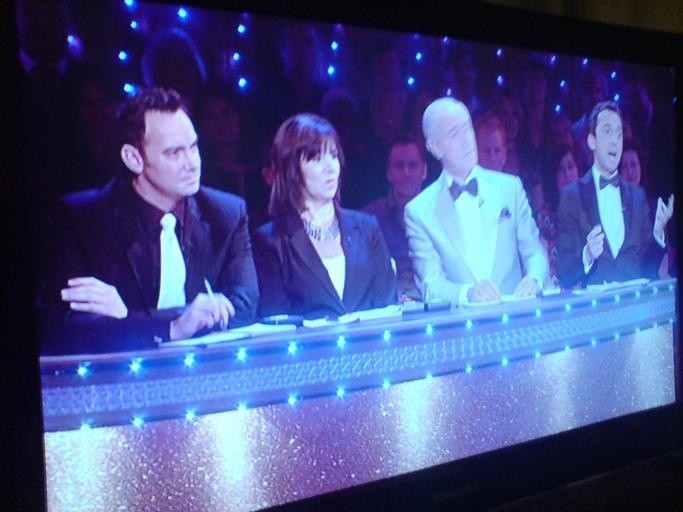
[156,213,187,310]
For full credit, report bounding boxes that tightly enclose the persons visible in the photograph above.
[52,86,258,354]
[14,1,656,304]
[556,102,675,289]
[247,114,399,322]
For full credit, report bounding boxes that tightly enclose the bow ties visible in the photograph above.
[449,179,478,202]
[599,174,621,188]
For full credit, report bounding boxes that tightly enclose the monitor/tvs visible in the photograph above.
[0,1,683,512]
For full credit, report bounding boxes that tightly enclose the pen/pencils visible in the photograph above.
[202,276,224,331]
